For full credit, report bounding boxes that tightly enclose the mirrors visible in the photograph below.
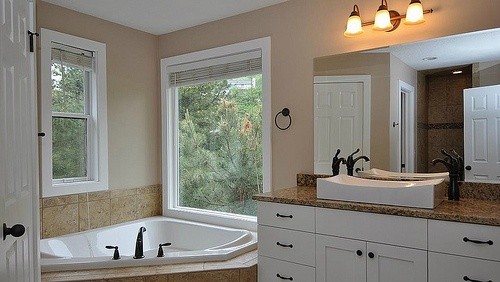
[312,29,500,184]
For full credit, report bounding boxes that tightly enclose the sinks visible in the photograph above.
[316,171,446,209]
[358,167,451,182]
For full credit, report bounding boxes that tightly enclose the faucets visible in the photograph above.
[347,148,369,177]
[431,157,461,202]
[441,147,464,163]
[132,226,147,260]
[331,148,347,175]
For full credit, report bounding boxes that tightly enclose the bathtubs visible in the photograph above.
[38,213,258,274]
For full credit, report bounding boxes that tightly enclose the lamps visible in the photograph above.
[344,0,433,38]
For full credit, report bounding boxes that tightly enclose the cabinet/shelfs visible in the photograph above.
[252,201,500,282]
